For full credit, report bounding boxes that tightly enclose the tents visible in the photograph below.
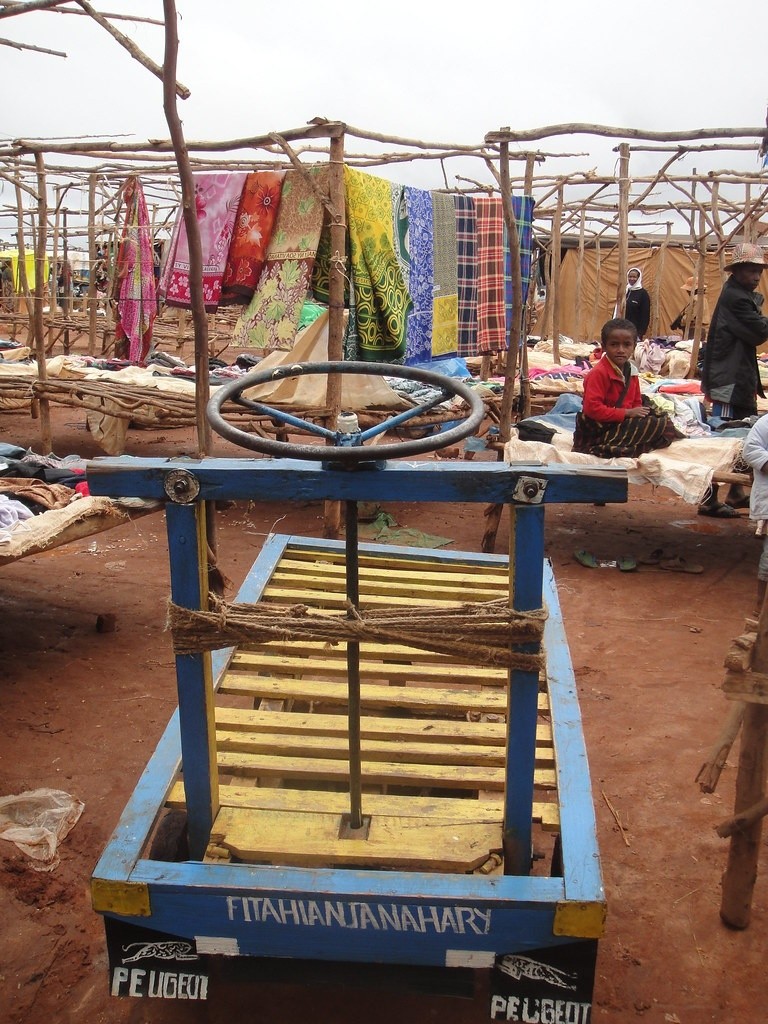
[530,234,768,364]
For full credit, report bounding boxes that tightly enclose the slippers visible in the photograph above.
[661,556,704,572]
[575,550,598,567]
[729,496,752,508]
[698,503,742,518]
[618,556,636,571]
[639,550,671,564]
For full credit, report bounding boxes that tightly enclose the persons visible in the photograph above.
[536,286,546,302]
[678,275,711,344]
[696,242,768,517]
[571,318,689,458]
[612,267,650,343]
[0,260,65,313]
[741,413,768,617]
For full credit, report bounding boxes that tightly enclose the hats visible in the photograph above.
[681,277,707,291]
[724,244,768,271]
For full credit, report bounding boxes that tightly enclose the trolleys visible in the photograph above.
[90,359,629,998]
